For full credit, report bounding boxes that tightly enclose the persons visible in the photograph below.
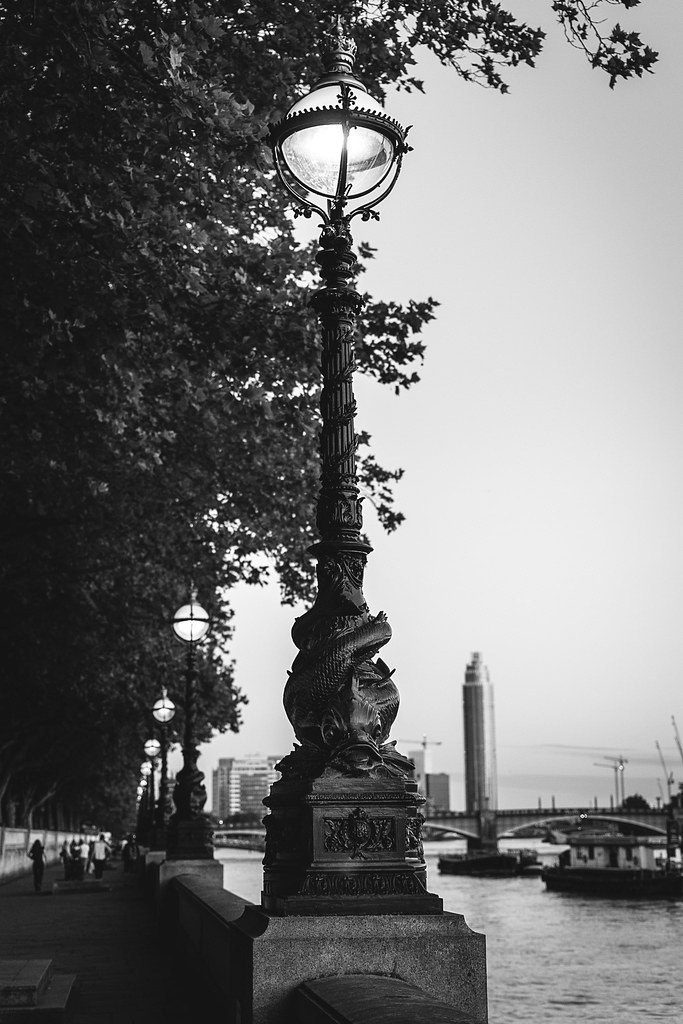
[61,835,137,883]
[27,838,46,891]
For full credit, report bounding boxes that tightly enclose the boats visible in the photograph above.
[539,835,682,897]
[437,845,541,877]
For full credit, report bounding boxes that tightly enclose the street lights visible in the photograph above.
[137,759,152,845]
[152,685,177,851]
[166,581,212,861]
[257,11,441,920]
[145,733,162,848]
